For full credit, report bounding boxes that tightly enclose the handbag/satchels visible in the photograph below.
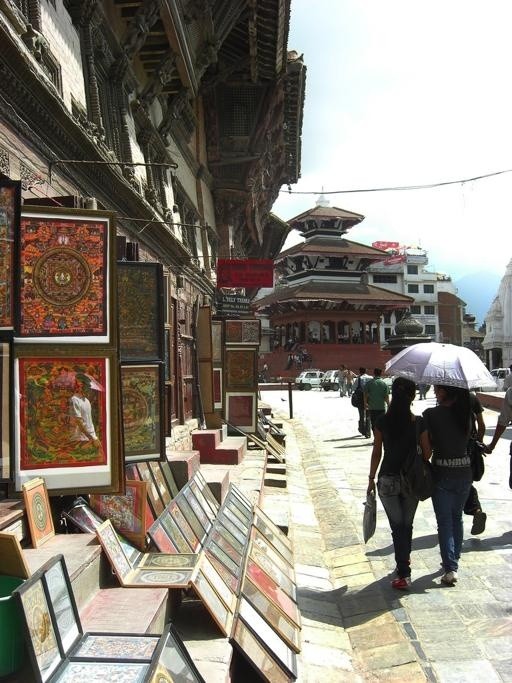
[472,441,484,481]
[412,463,431,501]
[362,495,377,544]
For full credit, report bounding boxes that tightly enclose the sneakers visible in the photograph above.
[441,572,459,585]
[392,578,410,588]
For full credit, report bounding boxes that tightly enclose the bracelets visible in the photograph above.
[369,475,375,479]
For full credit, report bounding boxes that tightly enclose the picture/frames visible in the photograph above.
[0,172,166,497]
[196,306,261,434]
[1,448,302,683]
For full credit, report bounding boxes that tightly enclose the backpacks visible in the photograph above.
[352,389,363,408]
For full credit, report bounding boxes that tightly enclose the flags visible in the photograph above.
[216,257,275,290]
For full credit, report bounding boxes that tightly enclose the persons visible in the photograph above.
[367,376,432,590]
[421,384,474,586]
[274,324,379,345]
[352,367,374,439]
[364,367,392,445]
[334,364,348,397]
[284,344,312,371]
[418,383,427,400]
[345,367,358,396]
[263,360,272,382]
[463,388,487,537]
[486,386,512,488]
[68,370,104,451]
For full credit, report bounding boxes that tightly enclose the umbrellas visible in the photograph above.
[383,339,499,393]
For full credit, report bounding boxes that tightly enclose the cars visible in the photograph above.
[481,368,511,391]
[295,367,324,390]
[321,369,358,390]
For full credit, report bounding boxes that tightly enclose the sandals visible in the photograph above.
[471,512,486,535]
